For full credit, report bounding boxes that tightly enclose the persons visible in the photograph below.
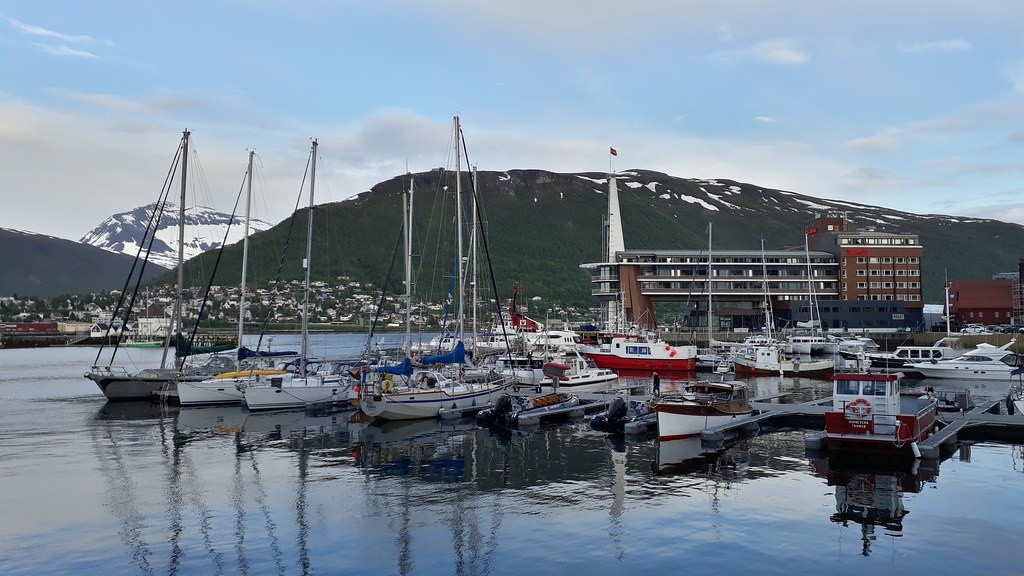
[611,147,617,156]
[653,372,660,398]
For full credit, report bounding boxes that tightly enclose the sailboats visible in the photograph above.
[81,124,234,403]
[176,108,880,435]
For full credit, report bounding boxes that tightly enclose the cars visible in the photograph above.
[958,323,1024,336]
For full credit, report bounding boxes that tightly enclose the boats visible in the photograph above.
[901,342,1024,381]
[836,339,971,376]
[820,322,942,452]
[651,423,760,474]
[810,444,944,557]
[651,354,755,440]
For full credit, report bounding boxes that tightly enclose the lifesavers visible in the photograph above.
[380,380,393,390]
[853,398,871,416]
